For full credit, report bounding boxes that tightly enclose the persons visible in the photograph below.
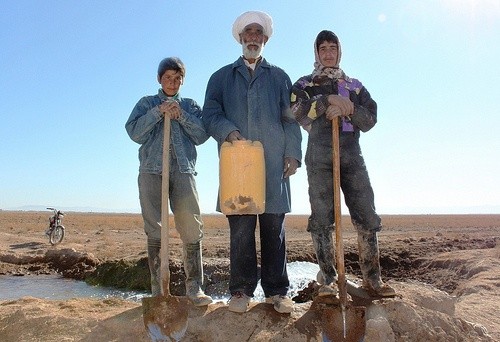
[125,56,213,306]
[291,30,396,295]
[202,11,302,312]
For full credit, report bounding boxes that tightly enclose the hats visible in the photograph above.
[232,11,273,44]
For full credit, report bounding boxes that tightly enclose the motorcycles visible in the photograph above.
[44,207,65,245]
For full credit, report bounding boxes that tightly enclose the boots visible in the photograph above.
[310,225,336,296]
[148,245,163,296]
[182,241,213,307]
[357,230,395,296]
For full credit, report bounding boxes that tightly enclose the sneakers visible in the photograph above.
[265,295,295,313]
[229,294,251,312]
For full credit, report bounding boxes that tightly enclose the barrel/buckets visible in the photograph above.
[220,138,265,215]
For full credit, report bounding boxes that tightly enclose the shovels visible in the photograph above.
[141,112,190,342]
[322,115,366,342]
